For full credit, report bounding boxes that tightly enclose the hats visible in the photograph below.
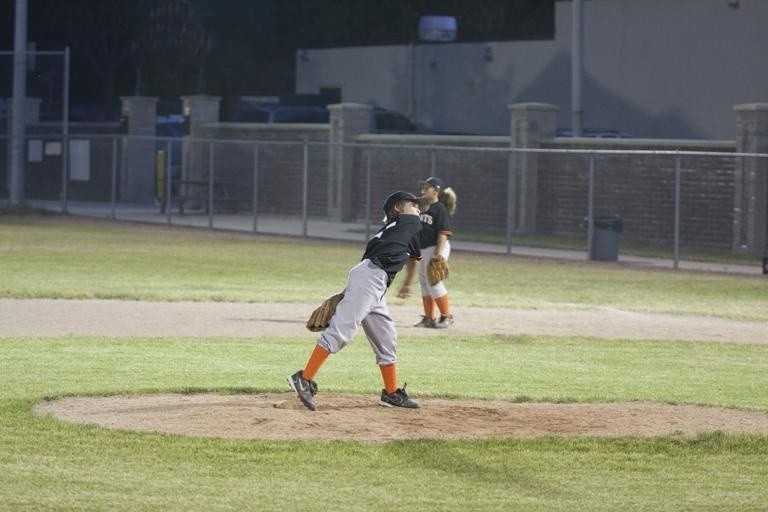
[384,191,424,215]
[418,176,445,192]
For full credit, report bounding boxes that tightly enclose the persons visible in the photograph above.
[287,191,423,411]
[414,177,455,329]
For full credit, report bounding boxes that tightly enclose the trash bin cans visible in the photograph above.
[588,217,622,261]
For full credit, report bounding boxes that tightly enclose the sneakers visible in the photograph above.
[287,371,317,411]
[378,388,420,409]
[414,318,436,327]
[434,315,454,327]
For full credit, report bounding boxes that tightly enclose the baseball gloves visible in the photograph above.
[306,294,346,331]
[427,257,450,285]
